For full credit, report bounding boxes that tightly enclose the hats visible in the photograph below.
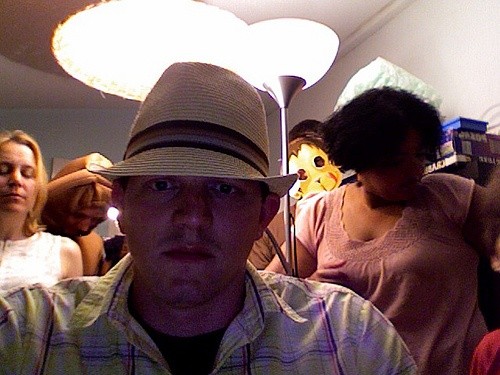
[86,61,298,202]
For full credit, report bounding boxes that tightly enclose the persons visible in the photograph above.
[0,63,420,375]
[248,120,342,270]
[37,152,114,276]
[102,234,130,269]
[0,129,82,291]
[265,86,500,375]
[467,164,500,375]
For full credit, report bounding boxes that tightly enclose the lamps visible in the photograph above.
[50,1,250,104]
[247,18,340,279]
